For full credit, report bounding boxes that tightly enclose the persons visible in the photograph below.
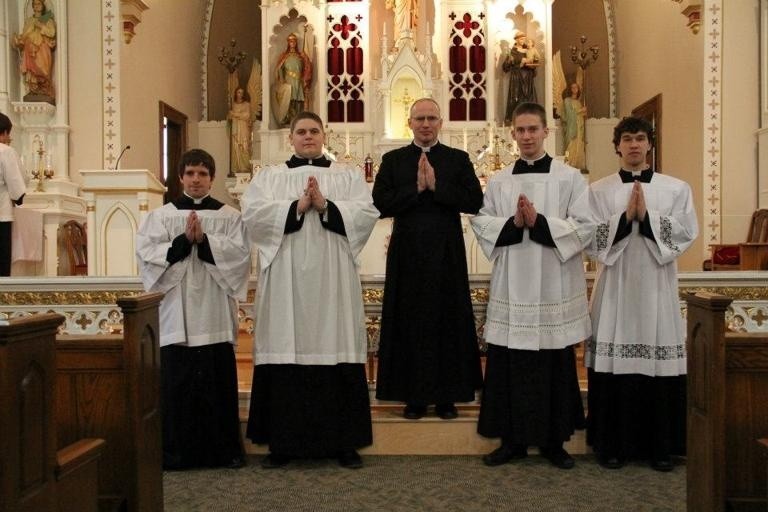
[373,99,484,420]
[385,0,421,54]
[501,33,542,125]
[138,148,253,470]
[514,38,540,69]
[584,117,696,468]
[240,110,375,470]
[0,113,27,278]
[273,33,314,127]
[560,81,590,175]
[12,0,57,104]
[228,86,253,175]
[470,103,594,470]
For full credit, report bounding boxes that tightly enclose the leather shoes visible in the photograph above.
[650,457,674,472]
[482,442,528,466]
[600,455,624,471]
[539,445,575,470]
[403,404,429,419]
[435,404,456,418]
[341,446,363,468]
[261,449,292,470]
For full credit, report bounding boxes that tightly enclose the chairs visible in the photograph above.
[703,208,768,270]
[64,219,88,275]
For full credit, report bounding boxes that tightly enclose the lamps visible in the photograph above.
[120,0,149,44]
[672,0,702,35]
